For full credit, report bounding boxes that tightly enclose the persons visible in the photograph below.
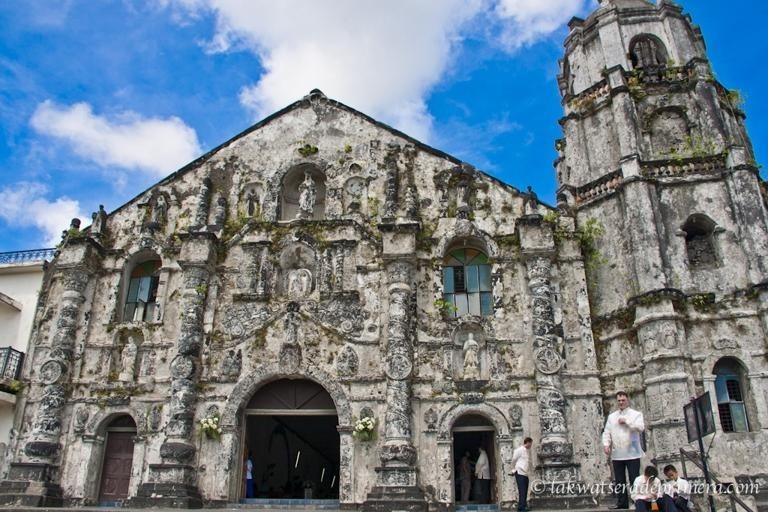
[245,188,261,216]
[508,438,532,510]
[522,186,538,215]
[296,169,316,217]
[95,204,108,232]
[659,463,691,511]
[474,444,490,504]
[302,474,316,500]
[247,460,253,499]
[602,391,645,509]
[462,334,481,379]
[460,450,472,505]
[632,466,663,511]
[120,336,138,378]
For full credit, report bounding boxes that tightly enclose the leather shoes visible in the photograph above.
[608,503,628,509]
